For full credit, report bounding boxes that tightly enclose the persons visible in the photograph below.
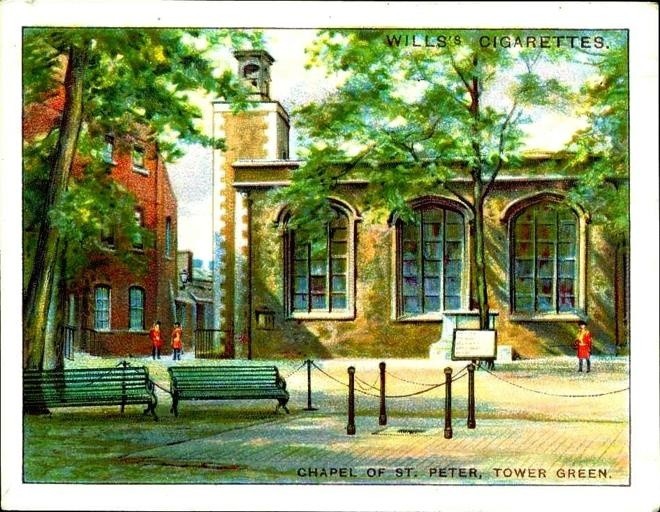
[574,319,593,373]
[170,322,183,360]
[149,320,161,361]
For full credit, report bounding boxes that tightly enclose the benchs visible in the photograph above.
[168,366,290,418]
[23,366,159,422]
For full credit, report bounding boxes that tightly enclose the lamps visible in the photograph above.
[178,269,189,290]
